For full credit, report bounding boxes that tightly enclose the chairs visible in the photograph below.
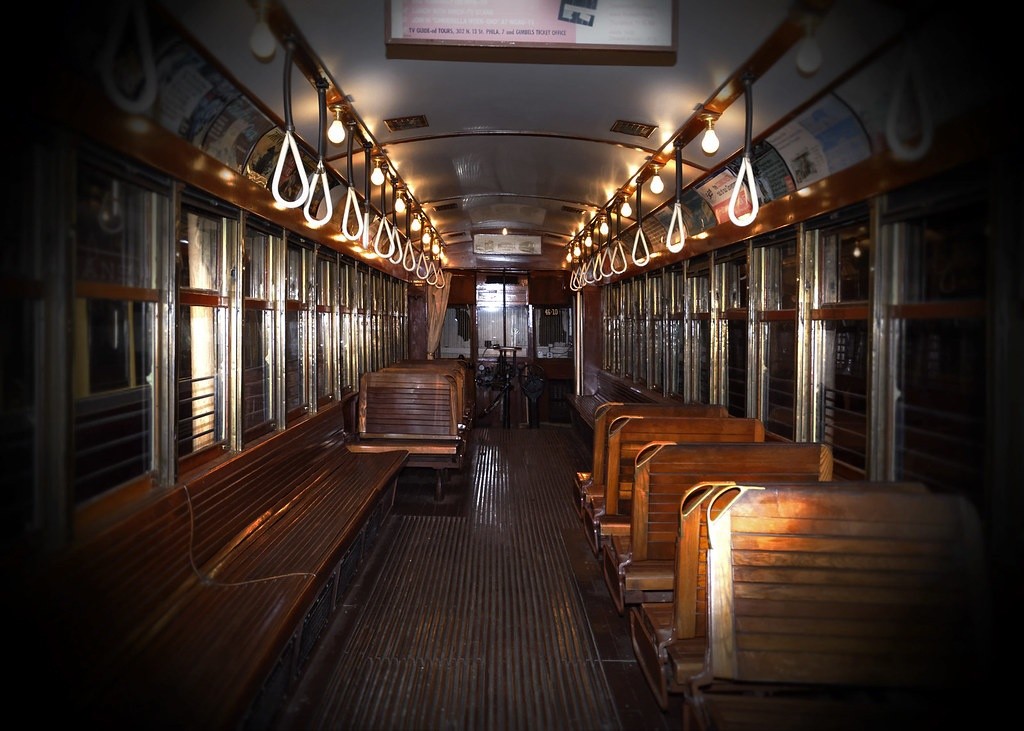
[571,402,1024,731]
[346,356,477,502]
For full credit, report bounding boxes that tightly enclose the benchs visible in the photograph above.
[564,368,679,434]
[23,387,410,731]
